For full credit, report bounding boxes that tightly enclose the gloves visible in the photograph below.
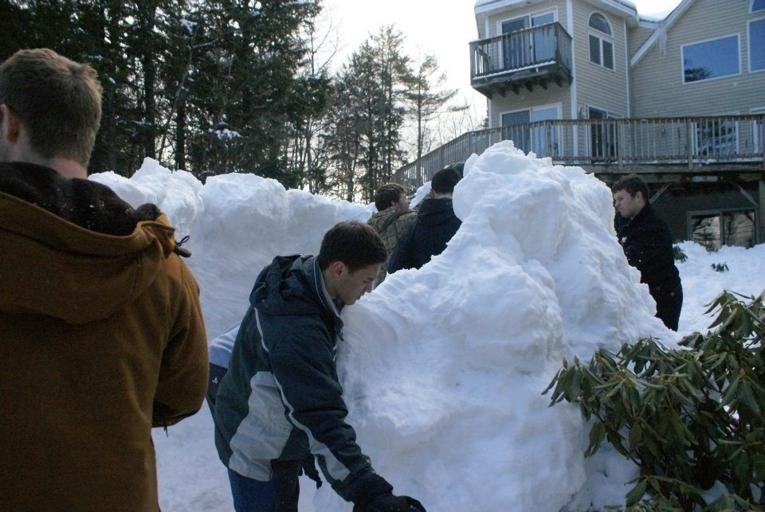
[352,493,425,512]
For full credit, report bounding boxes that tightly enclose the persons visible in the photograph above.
[210,216,427,510]
[387,170,467,274]
[363,184,421,264]
[0,44,213,510]
[608,171,682,332]
